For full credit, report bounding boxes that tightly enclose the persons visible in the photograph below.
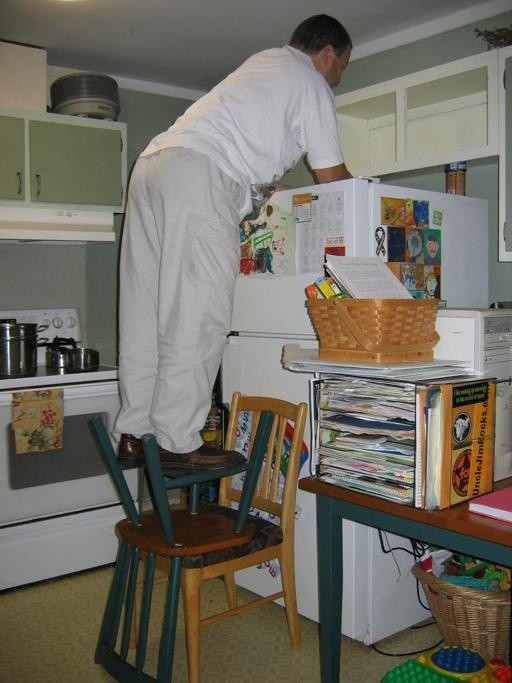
[108,6,358,479]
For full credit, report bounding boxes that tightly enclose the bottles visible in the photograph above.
[444,160,467,195]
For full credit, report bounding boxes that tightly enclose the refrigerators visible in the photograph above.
[218,176,489,647]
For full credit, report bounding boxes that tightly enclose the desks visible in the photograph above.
[298,474,512,683]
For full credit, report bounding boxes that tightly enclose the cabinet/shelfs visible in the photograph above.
[1,106,128,239]
[333,44,512,265]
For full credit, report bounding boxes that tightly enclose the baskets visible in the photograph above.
[304,297,443,366]
[410,552,511,662]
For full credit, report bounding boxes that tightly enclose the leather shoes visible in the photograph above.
[115,433,247,473]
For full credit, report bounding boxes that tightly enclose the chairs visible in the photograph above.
[89,391,305,683]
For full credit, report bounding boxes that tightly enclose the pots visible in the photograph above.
[0,319,99,378]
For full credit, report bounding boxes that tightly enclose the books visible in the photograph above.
[315,373,493,513]
[305,252,412,302]
[466,485,511,522]
